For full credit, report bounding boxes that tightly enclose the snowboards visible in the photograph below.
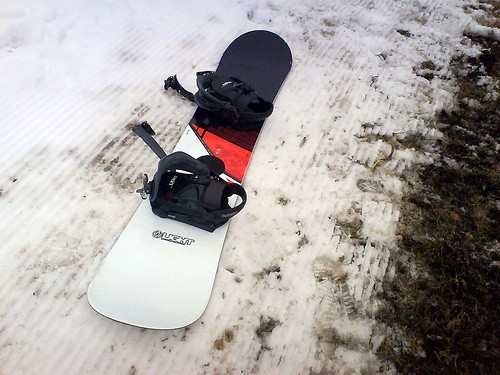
[84,30,293,331]
